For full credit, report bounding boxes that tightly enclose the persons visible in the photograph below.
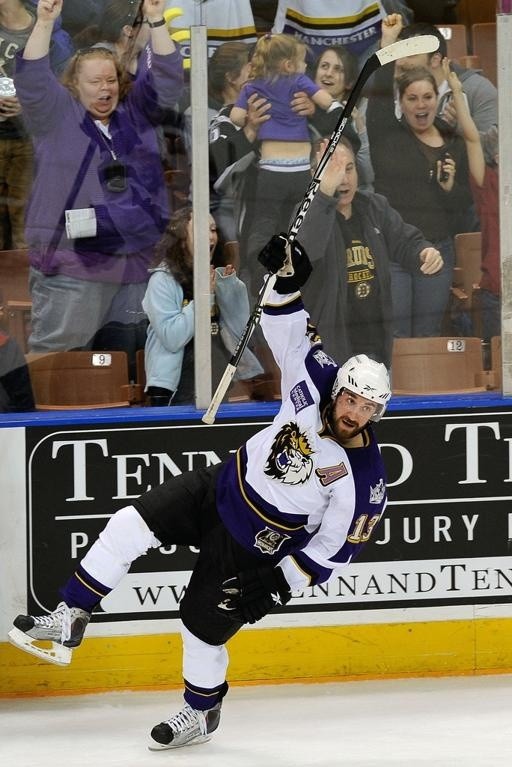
[11,232,392,747]
[1,0,499,414]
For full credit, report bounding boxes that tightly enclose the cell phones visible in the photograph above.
[440,153,451,186]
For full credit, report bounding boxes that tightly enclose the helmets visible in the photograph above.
[330,352,395,424]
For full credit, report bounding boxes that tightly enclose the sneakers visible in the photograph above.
[5,602,92,668]
[144,706,224,753]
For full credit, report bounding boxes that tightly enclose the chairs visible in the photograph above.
[136,350,253,403]
[23,350,130,411]
[456,0,496,41]
[0,249,31,307]
[450,231,485,336]
[473,23,497,88]
[491,336,502,392]
[8,299,33,353]
[390,336,486,396]
[432,24,467,59]
[454,56,481,75]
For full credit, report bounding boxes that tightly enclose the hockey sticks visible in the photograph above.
[199,32,440,426]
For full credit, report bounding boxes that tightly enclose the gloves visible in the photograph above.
[256,232,314,295]
[217,563,293,626]
